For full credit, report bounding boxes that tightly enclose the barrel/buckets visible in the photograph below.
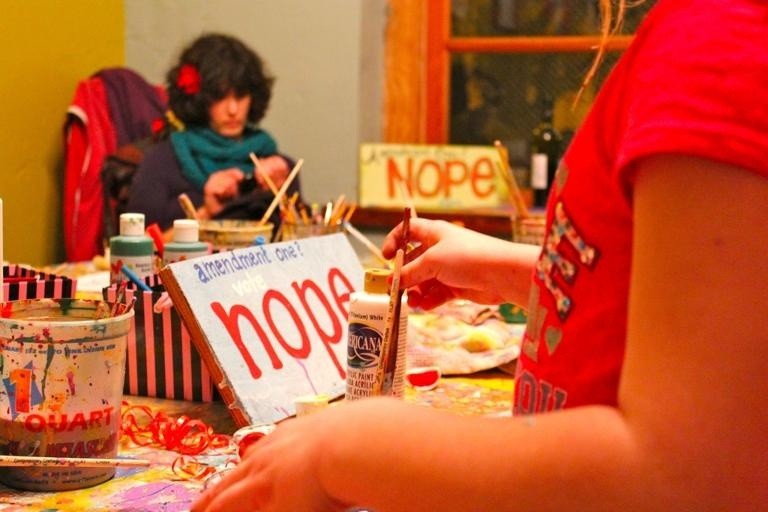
[1,296,136,492]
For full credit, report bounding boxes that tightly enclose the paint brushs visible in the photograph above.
[112,280,137,319]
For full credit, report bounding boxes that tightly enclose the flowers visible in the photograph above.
[175,64,201,97]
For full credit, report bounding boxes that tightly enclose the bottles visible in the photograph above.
[346,269,408,404]
[531,96,563,207]
[109,213,208,284]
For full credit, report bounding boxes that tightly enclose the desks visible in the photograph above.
[0,394,241,512]
[321,202,548,252]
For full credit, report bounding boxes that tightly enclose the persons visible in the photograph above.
[187,1,767,512]
[125,34,300,241]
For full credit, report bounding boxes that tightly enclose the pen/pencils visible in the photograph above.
[495,140,528,219]
[0,455,152,468]
[121,264,152,291]
[371,207,412,396]
[250,152,356,226]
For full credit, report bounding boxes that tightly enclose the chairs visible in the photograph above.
[64,65,171,263]
